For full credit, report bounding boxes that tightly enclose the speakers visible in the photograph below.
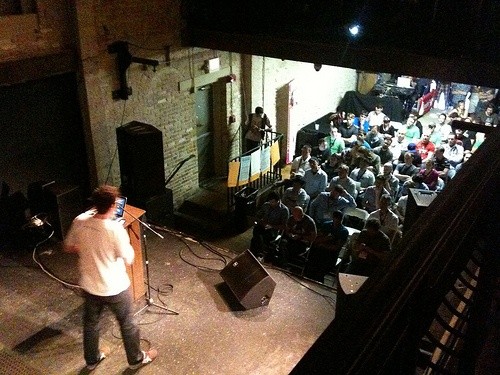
[403,187,438,236]
[219,249,276,310]
[336,273,368,315]
[116,120,166,200]
[37,171,82,242]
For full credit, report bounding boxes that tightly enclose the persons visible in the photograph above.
[242,107,273,156]
[250,72,500,286]
[63,185,157,371]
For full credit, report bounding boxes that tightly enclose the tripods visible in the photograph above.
[124,212,180,319]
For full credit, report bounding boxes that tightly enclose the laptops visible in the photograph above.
[114,196,127,221]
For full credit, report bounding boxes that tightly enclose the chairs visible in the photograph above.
[340,207,368,236]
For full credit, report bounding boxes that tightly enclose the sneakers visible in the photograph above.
[86,346,110,370]
[128,350,158,369]
